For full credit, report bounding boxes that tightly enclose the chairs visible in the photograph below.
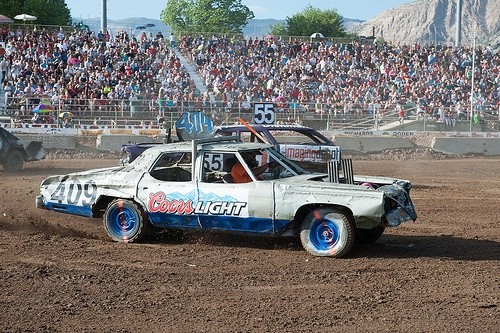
[220,158,238,184]
[151,167,190,181]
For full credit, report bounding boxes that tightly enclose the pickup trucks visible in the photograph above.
[120,125,343,172]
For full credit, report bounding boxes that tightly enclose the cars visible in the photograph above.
[1,126,46,174]
[36,136,418,257]
